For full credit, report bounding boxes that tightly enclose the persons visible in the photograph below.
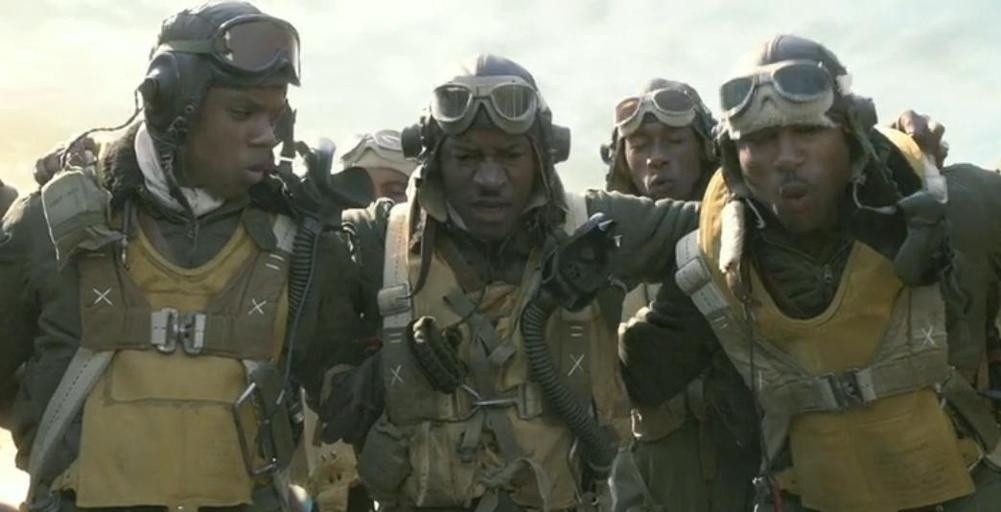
[286,124,420,511]
[0,1,385,512]
[31,51,948,512]
[614,27,1001,511]
[597,74,720,510]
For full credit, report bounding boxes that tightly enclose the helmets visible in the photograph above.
[608,78,713,184]
[418,53,553,204]
[144,0,289,151]
[720,34,856,186]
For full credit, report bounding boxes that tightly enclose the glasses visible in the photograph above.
[330,128,411,166]
[720,61,833,121]
[211,14,302,85]
[612,88,698,135]
[432,74,541,135]
